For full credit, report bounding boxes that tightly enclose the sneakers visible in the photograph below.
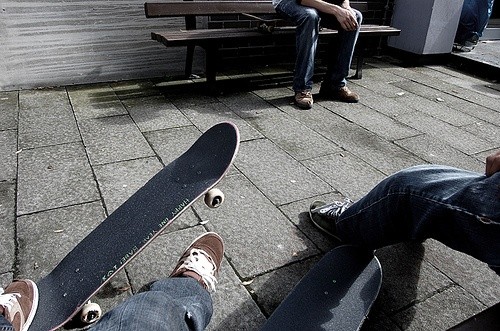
[293,88,313,109]
[319,82,360,104]
[401,243,424,255]
[0,279,39,331]
[309,200,373,252]
[168,230,225,299]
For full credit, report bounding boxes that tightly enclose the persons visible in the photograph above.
[272,0,363,110]
[307,150,500,279]
[0,231,225,330]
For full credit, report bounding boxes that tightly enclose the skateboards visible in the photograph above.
[27,120,241,330]
[259,245,384,331]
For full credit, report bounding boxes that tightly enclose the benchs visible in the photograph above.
[144,1,402,80]
